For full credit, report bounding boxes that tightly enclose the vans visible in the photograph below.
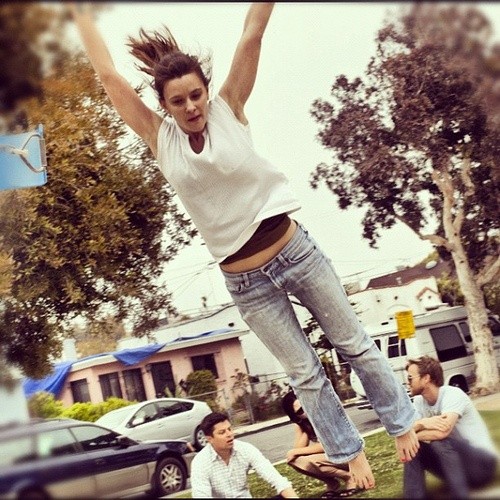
[349,305,499,407]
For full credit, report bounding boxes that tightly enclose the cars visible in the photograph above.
[96,398,213,451]
[1,422,187,499]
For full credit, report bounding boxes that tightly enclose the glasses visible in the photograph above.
[407,374,423,382]
[295,407,304,416]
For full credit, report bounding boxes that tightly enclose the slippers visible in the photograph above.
[320,491,339,499]
[339,488,361,497]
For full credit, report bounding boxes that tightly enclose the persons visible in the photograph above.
[403,356,500,500]
[190,412,299,498]
[282,391,358,499]
[70,2,420,489]
